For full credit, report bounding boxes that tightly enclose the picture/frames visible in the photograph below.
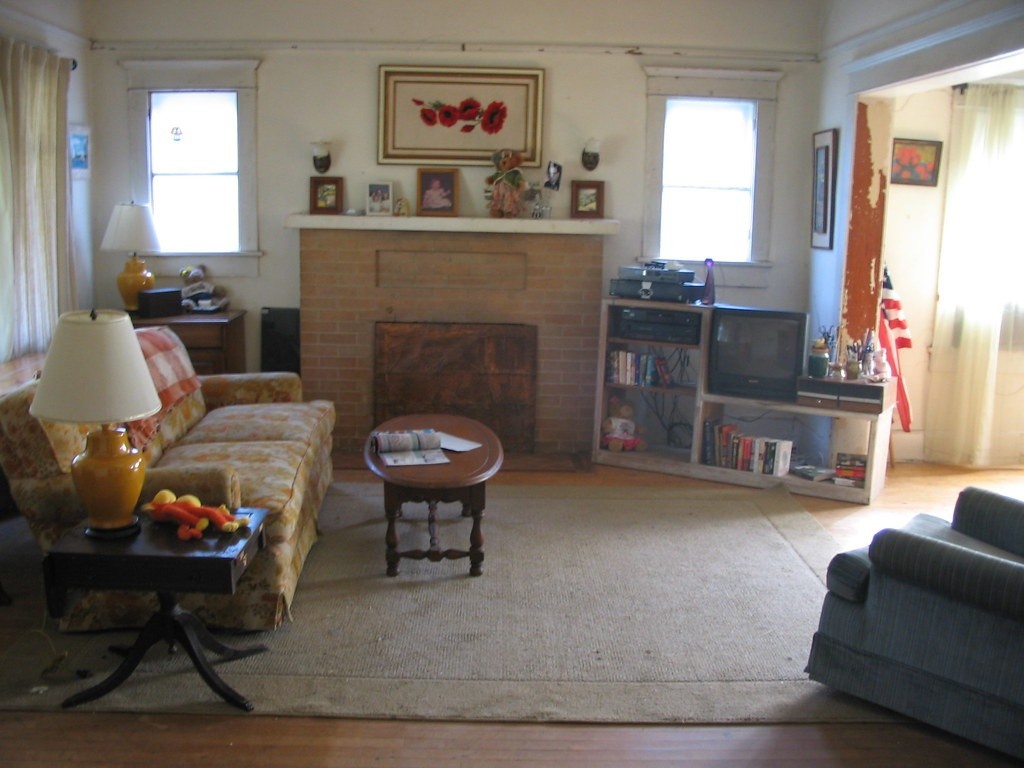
[374,62,546,170]
[68,124,93,180]
[309,175,344,215]
[416,167,461,217]
[809,126,838,251]
[570,178,606,220]
[366,181,393,216]
[890,137,944,187]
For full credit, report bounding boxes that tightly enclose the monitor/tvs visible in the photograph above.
[707,305,811,402]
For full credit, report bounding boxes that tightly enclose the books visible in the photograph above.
[704,416,794,476]
[655,356,673,386]
[833,452,868,489]
[368,427,451,466]
[607,349,658,387]
[791,465,835,481]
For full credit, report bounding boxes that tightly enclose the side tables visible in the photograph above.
[129,307,247,375]
[42,496,276,716]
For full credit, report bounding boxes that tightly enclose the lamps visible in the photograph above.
[580,138,601,170]
[311,142,331,174]
[25,304,165,542]
[99,198,163,314]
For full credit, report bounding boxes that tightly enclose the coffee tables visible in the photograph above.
[361,410,504,578]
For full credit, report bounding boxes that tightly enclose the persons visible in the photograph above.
[422,179,453,208]
[370,189,390,212]
[544,161,561,191]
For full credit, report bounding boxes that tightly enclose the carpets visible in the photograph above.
[0,481,913,728]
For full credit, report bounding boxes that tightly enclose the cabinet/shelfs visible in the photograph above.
[598,296,890,505]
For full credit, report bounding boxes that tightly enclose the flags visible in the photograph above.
[880,261,912,432]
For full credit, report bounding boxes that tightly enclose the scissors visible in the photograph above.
[817,325,834,342]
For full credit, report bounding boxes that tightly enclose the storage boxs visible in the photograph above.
[839,374,899,414]
[138,287,184,318]
[795,373,838,410]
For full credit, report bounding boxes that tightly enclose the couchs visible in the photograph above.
[803,482,1024,761]
[0,322,339,638]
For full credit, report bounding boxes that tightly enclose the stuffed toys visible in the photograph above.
[180,263,229,311]
[141,489,249,540]
[601,395,648,452]
[483,148,527,218]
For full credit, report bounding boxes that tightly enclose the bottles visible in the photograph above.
[846,360,860,380]
[809,346,829,377]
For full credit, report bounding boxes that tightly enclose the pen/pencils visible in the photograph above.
[853,327,876,363]
